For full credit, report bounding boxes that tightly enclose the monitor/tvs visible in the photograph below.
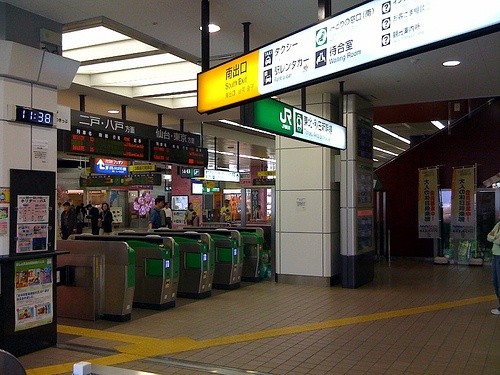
[171,195,190,211]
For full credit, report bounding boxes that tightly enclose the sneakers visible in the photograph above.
[491,308,500,315]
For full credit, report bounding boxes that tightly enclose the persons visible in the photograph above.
[487,219,500,315]
[148,197,164,229]
[71,199,262,235]
[60,201,75,240]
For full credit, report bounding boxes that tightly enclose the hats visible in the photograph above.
[63,202,70,206]
[86,204,90,210]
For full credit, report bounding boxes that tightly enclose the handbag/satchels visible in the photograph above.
[98,220,102,227]
